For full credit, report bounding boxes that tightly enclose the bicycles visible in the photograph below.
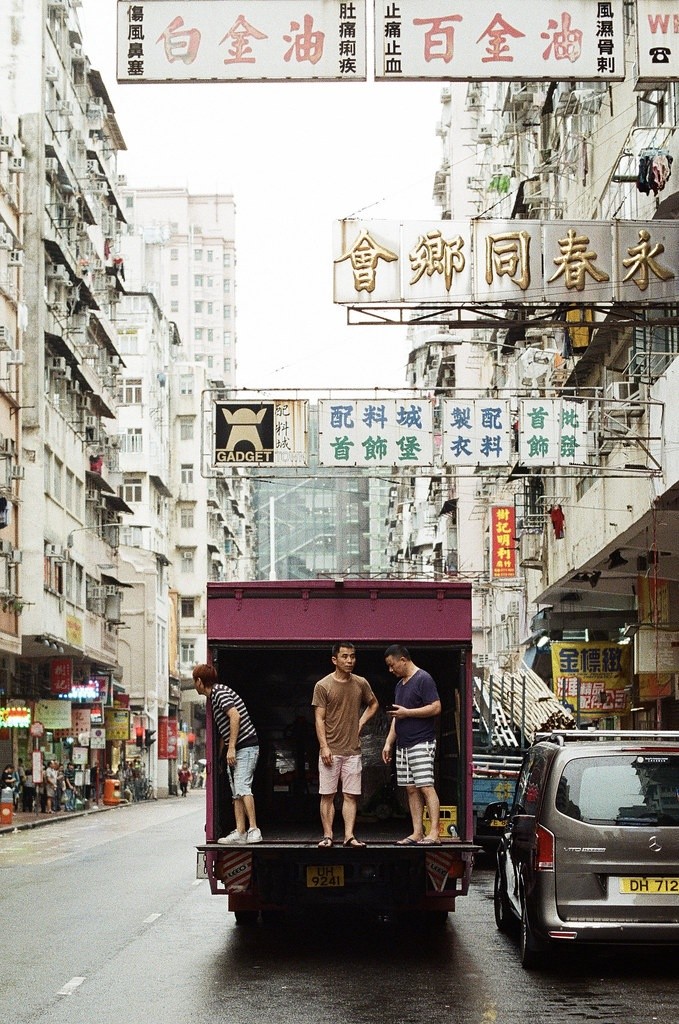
[146,779,153,799]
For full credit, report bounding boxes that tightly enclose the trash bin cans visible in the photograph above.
[0,789,13,824]
[104,779,121,805]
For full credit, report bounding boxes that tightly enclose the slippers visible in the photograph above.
[318,837,333,848]
[343,837,368,848]
[413,839,442,846]
[394,837,417,846]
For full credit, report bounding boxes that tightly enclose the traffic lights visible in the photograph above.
[135,727,142,747]
[145,729,156,746]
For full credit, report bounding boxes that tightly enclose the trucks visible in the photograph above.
[472,720,518,866]
[196,573,474,927]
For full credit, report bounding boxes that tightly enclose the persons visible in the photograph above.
[103,763,114,779]
[64,763,76,812]
[193,664,262,844]
[382,643,443,845]
[178,764,191,797]
[198,765,206,788]
[1,758,34,813]
[312,642,379,848]
[125,761,137,778]
[190,762,199,789]
[90,760,104,801]
[117,765,126,782]
[36,759,64,814]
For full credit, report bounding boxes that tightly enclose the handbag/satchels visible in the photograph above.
[61,792,70,803]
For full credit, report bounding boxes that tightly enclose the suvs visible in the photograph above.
[482,736,679,970]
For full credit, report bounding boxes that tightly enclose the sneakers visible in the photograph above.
[246,828,262,843]
[218,829,247,844]
